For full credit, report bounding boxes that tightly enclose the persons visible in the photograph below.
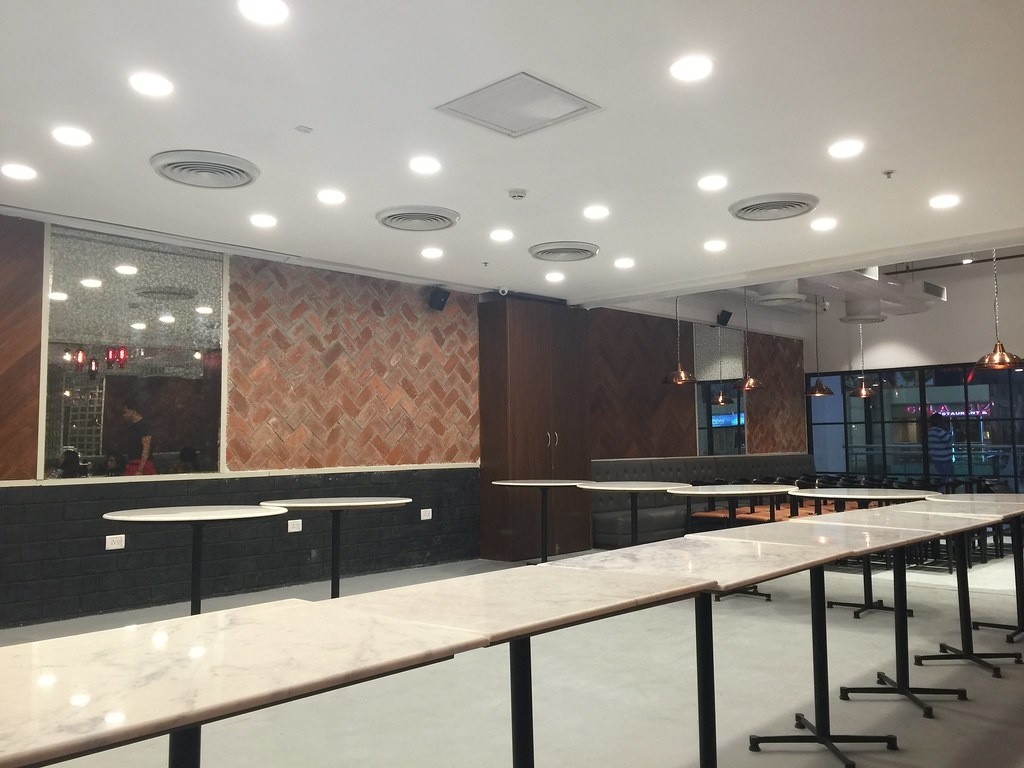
[928,413,955,474]
[107,452,126,476]
[180,447,201,472]
[122,402,156,475]
[59,450,81,478]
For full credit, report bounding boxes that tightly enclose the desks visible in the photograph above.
[1,479,1024,768]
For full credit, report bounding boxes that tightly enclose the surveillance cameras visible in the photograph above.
[821,302,829,311]
[499,287,508,295]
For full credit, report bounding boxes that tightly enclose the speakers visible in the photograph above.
[718,310,733,326]
[429,287,450,311]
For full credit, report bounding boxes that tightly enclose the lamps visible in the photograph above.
[976,249,1024,369]
[849,323,876,398]
[708,327,733,406]
[732,286,766,391]
[661,296,698,385]
[802,295,834,397]
[63,343,128,380]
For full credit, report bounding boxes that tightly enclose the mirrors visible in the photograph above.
[34,220,228,480]
[691,319,746,457]
